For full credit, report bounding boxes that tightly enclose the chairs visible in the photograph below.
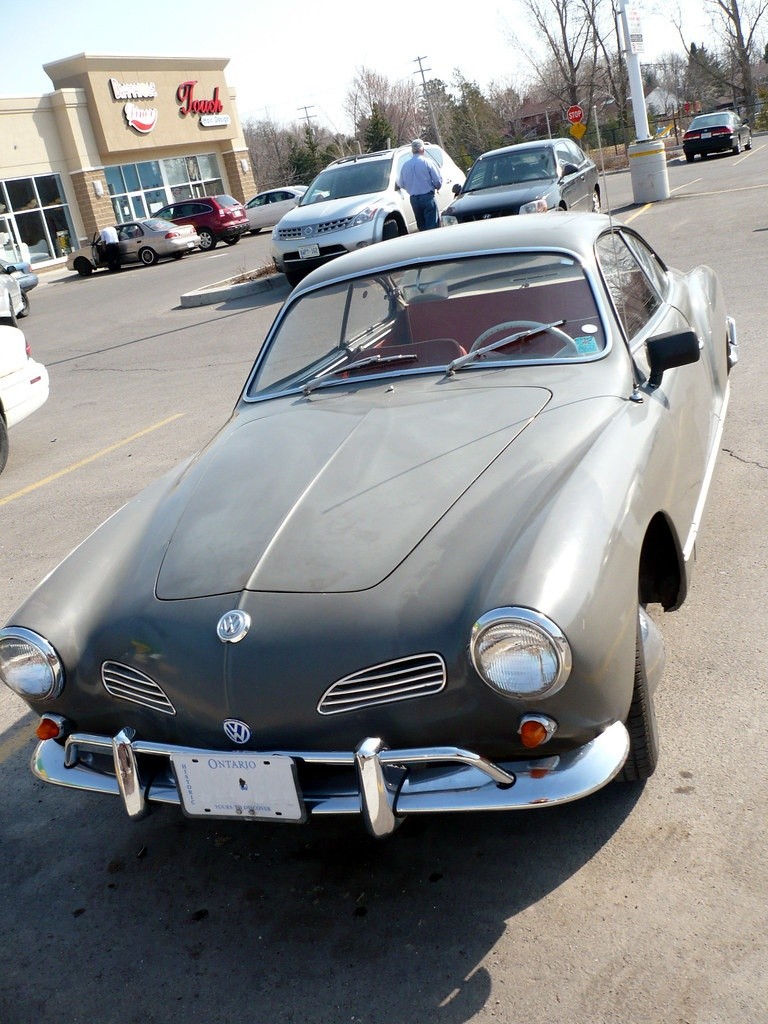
[270,197,277,202]
[492,158,548,182]
[346,339,461,378]
[488,311,644,355]
[281,194,289,200]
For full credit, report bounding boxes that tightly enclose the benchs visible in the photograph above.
[404,270,647,366]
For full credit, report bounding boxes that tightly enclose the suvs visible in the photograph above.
[269,139,467,287]
[150,194,250,251]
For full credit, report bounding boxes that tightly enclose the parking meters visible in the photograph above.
[567,105,585,124]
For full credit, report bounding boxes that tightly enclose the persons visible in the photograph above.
[101,225,122,272]
[397,139,442,231]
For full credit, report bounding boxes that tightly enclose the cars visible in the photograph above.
[66,217,202,278]
[0,210,735,844]
[0,259,41,327]
[440,138,602,230]
[0,325,50,476]
[242,185,330,234]
[683,110,752,163]
